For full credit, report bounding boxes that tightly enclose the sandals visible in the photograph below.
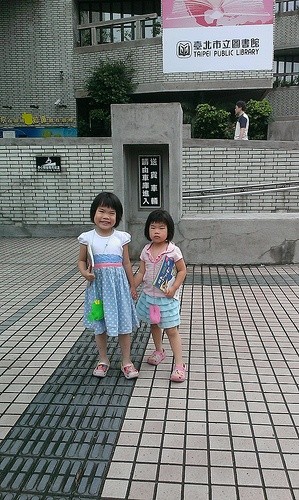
[169,364,186,382]
[120,361,139,379]
[147,347,167,365]
[93,360,111,377]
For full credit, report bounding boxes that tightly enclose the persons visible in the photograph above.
[233,100,250,140]
[77,193,139,380]
[131,210,187,383]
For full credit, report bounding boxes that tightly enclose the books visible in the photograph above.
[84,242,95,274]
[153,256,180,301]
